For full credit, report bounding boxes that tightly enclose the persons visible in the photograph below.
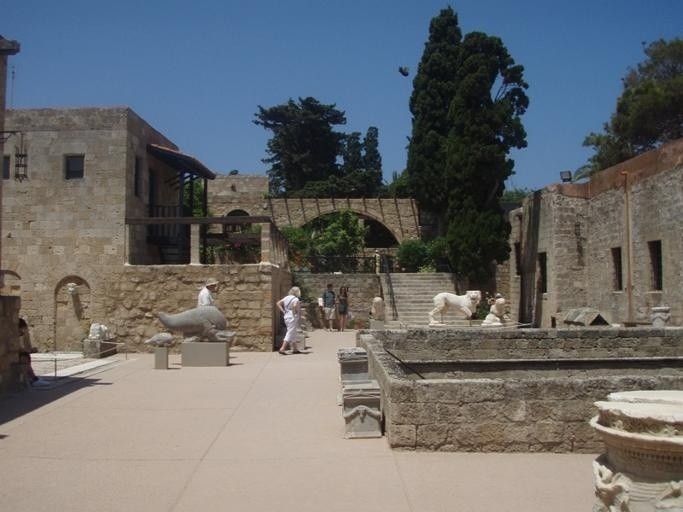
[321,283,336,333]
[275,286,302,356]
[196,277,219,307]
[18,316,50,387]
[337,285,349,332]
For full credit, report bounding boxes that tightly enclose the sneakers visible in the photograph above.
[32,380,50,387]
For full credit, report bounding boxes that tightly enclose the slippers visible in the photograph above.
[292,351,300,354]
[279,352,287,355]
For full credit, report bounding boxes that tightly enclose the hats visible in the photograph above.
[206,278,218,286]
